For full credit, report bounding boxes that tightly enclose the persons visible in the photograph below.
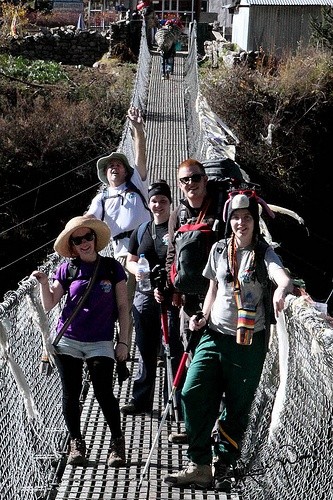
[154,159,233,442]
[164,188,294,493]
[29,217,130,467]
[121,179,172,412]
[84,107,165,366]
[161,19,175,79]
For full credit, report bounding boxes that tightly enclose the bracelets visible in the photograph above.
[117,342,129,348]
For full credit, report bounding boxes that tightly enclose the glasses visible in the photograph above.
[228,190,258,199]
[179,174,206,185]
[70,231,94,246]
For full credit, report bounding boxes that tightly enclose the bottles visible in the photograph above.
[236,293,256,345]
[137,254,151,292]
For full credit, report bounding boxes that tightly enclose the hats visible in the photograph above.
[53,217,111,258]
[96,152,134,186]
[148,179,171,204]
[223,194,275,283]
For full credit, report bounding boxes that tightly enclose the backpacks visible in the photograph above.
[170,198,218,291]
[180,156,261,226]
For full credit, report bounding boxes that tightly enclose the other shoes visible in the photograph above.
[168,431,187,443]
[155,356,164,367]
[160,405,182,420]
[162,70,169,79]
[120,399,153,414]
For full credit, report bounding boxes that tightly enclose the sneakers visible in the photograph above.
[213,461,232,490]
[67,438,86,464]
[163,459,212,486]
[107,443,126,466]
